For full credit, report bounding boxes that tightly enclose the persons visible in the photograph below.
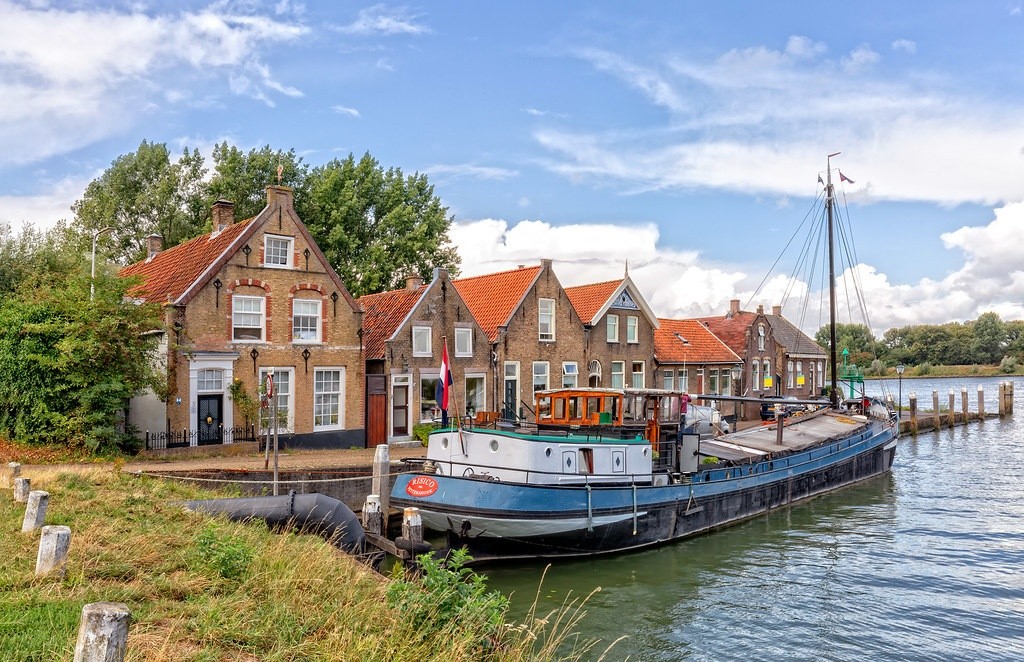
[465,403,475,415]
[862,397,870,416]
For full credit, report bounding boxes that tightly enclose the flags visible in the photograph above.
[435,339,451,425]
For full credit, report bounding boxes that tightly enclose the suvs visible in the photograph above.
[759,395,804,421]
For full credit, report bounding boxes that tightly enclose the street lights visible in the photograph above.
[895,364,904,420]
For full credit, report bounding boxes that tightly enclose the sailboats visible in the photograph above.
[383,155,901,566]
